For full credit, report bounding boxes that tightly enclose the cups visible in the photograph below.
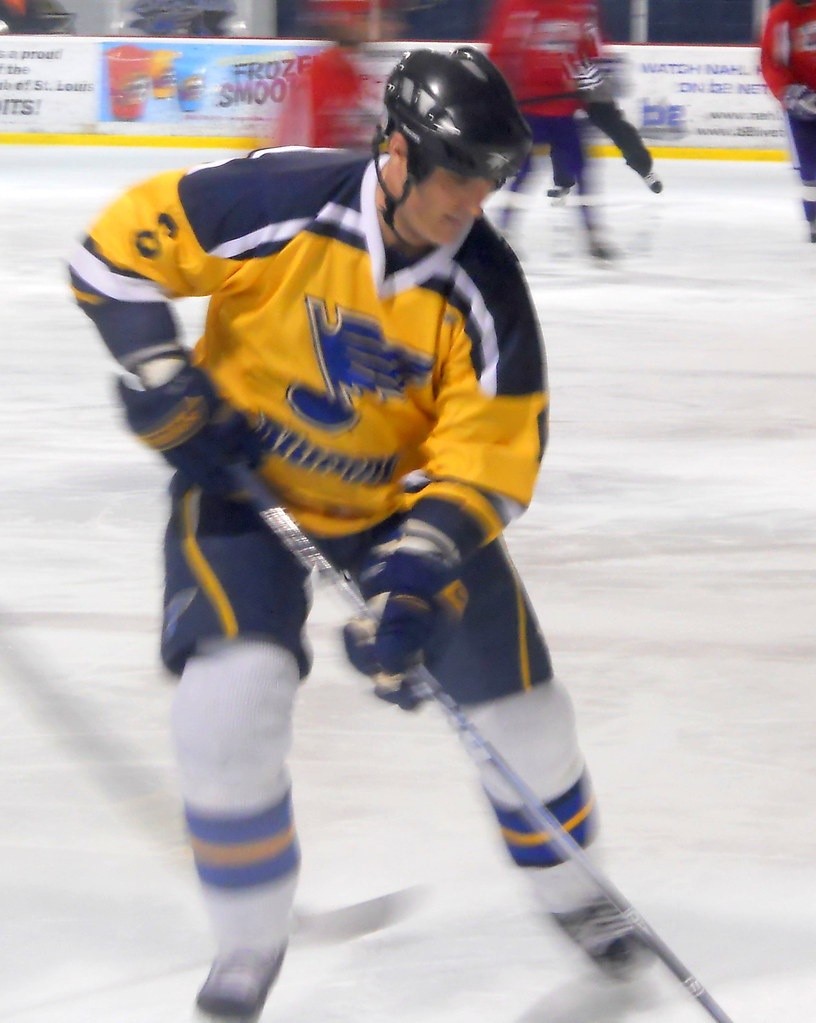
[103,48,205,121]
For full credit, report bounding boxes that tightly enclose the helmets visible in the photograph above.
[383,46,533,180]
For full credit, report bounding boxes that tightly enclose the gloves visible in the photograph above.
[574,64,613,104]
[345,495,482,710]
[118,364,259,495]
[786,84,816,121]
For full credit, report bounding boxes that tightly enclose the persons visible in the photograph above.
[67,46,652,1023]
[272,0,663,269]
[758,0,816,241]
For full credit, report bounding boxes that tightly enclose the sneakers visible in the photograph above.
[644,173,663,194]
[548,185,569,206]
[553,895,653,980]
[197,929,288,1023]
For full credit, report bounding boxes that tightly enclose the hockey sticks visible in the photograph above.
[226,452,733,1023]
[516,89,577,105]
